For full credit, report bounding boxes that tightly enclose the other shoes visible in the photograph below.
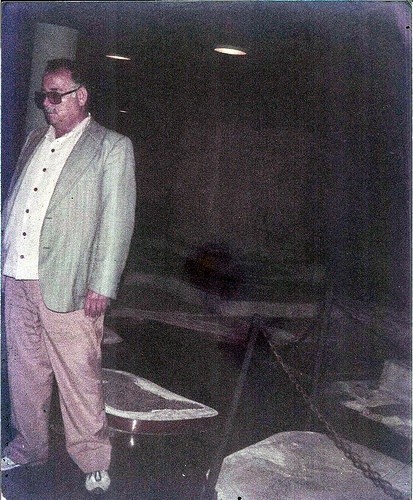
[84,470,110,496]
[0,455,21,471]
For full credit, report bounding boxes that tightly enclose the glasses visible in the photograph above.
[34,85,81,105]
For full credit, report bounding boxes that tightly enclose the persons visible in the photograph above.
[0,56,136,496]
